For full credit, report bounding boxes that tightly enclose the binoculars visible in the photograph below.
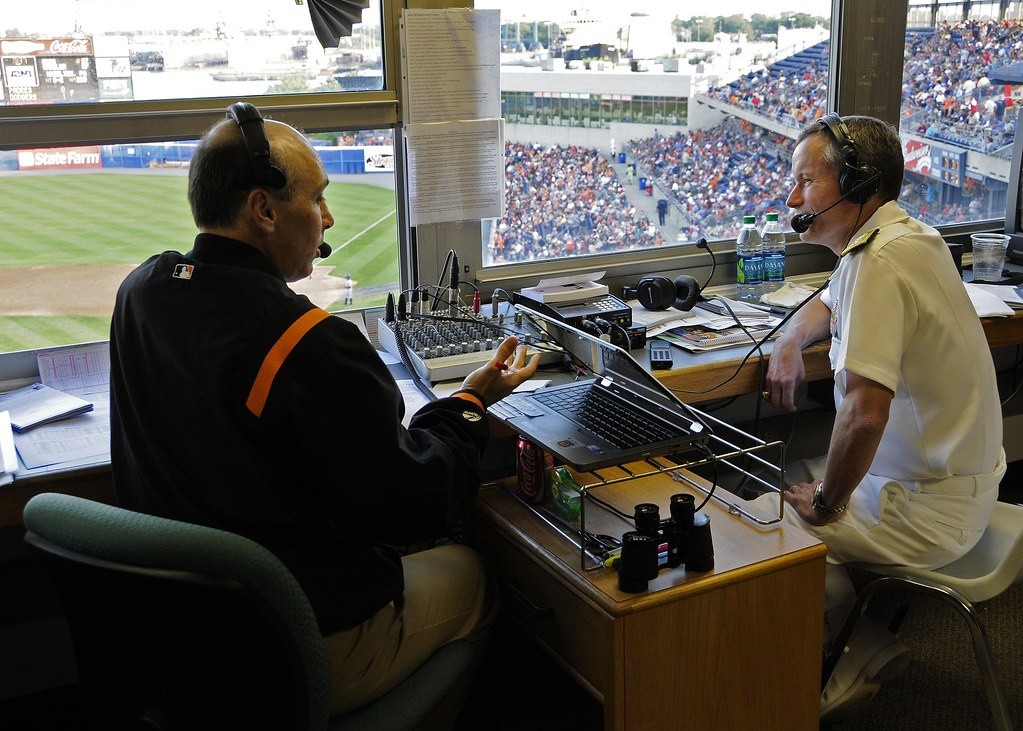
[618,493,715,593]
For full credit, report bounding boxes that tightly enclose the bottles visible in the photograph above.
[736,216,763,299]
[761,213,786,293]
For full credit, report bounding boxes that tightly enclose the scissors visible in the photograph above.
[542,506,621,560]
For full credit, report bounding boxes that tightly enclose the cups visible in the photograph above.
[970,233,1011,282]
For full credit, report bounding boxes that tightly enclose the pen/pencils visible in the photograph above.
[494,362,520,372]
[736,300,787,315]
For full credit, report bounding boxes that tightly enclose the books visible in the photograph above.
[520,271,786,354]
[0,382,95,433]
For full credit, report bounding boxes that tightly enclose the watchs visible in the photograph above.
[810,482,846,514]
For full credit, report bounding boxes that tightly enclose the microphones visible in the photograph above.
[791,172,881,233]
[318,242,332,259]
[695,238,715,293]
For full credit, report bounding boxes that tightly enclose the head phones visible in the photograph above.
[620,275,700,311]
[225,102,288,192]
[815,111,881,204]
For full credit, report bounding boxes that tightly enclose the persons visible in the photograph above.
[343,274,352,305]
[740,111,1007,718]
[108,96,541,731]
[709,17,1023,155]
[337,132,393,146]
[481,117,988,265]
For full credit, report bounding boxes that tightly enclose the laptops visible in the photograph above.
[486,304,714,472]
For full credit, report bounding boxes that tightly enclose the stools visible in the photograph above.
[821,497,1022,731]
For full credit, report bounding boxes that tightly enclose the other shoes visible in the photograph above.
[818,614,911,724]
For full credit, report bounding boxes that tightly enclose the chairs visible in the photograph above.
[710,25,1023,198]
[20,491,472,731]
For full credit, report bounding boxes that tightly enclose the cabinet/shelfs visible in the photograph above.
[469,455,829,731]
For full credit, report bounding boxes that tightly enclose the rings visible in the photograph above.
[761,392,771,399]
